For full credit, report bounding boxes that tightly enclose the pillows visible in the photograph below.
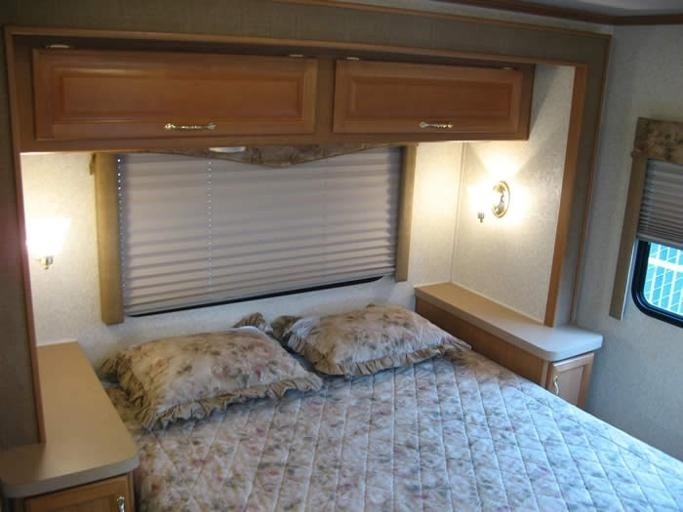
[269,305,472,383]
[96,311,324,430]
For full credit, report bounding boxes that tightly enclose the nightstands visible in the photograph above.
[2,340,141,512]
[416,282,603,410]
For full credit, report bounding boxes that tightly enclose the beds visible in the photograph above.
[99,333,681,512]
